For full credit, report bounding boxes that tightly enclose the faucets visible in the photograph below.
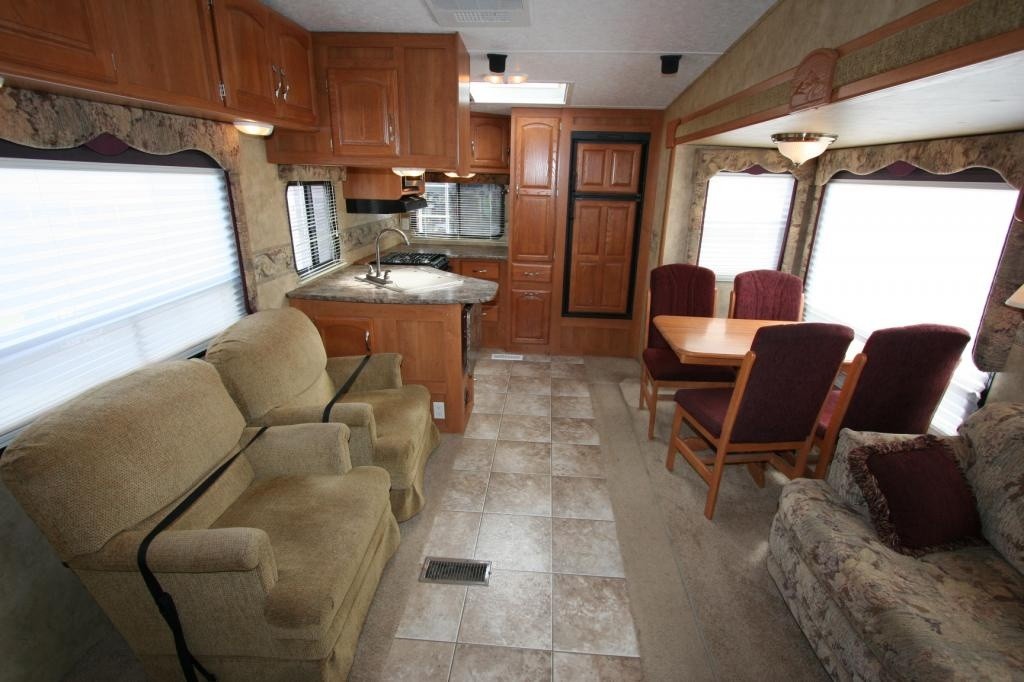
[375,227,410,277]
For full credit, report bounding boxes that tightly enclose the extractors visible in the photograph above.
[345,196,427,213]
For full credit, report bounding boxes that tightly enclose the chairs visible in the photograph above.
[666,322,854,519]
[728,269,804,322]
[638,263,716,439]
[783,324,971,480]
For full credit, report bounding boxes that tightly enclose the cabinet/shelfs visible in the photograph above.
[289,299,474,436]
[353,240,507,348]
[0,0,471,175]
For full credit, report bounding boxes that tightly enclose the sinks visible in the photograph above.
[354,268,464,293]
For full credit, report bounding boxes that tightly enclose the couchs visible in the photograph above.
[206,308,440,522]
[1,359,400,682]
[763,401,1024,682]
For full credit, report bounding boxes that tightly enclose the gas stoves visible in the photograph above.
[368,251,451,272]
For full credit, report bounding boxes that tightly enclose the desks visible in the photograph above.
[652,314,868,364]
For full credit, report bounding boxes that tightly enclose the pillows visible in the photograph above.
[847,433,983,559]
